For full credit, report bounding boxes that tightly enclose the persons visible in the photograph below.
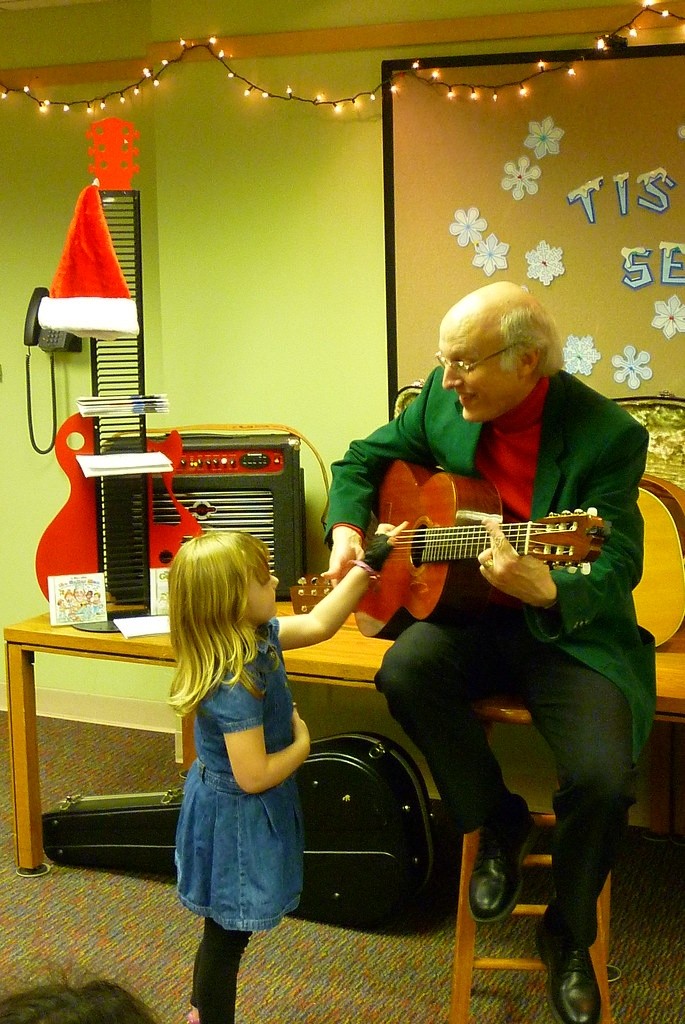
[165,519,409,1024]
[0,959,163,1024]
[321,280,655,1024]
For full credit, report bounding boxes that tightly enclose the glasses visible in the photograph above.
[435,343,519,373]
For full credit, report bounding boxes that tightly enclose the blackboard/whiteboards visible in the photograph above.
[378,42,684,493]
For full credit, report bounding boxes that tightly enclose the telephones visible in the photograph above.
[24,287,82,353]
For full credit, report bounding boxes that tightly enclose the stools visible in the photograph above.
[448,698,614,1024]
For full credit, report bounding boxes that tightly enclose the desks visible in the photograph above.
[4,600,685,878]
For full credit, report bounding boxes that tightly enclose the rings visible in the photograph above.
[483,559,493,568]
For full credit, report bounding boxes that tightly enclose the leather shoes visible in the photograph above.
[467,794,542,925]
[534,900,602,1024]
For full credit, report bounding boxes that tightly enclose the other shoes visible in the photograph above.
[186,1005,200,1024]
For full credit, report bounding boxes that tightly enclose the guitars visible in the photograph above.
[352,458,608,643]
[33,114,205,636]
[288,477,685,651]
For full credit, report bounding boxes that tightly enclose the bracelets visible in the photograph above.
[349,559,375,572]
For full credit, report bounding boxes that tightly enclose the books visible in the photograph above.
[113,616,171,639]
[75,452,173,478]
[76,393,170,418]
[149,567,171,615]
[47,574,109,627]
